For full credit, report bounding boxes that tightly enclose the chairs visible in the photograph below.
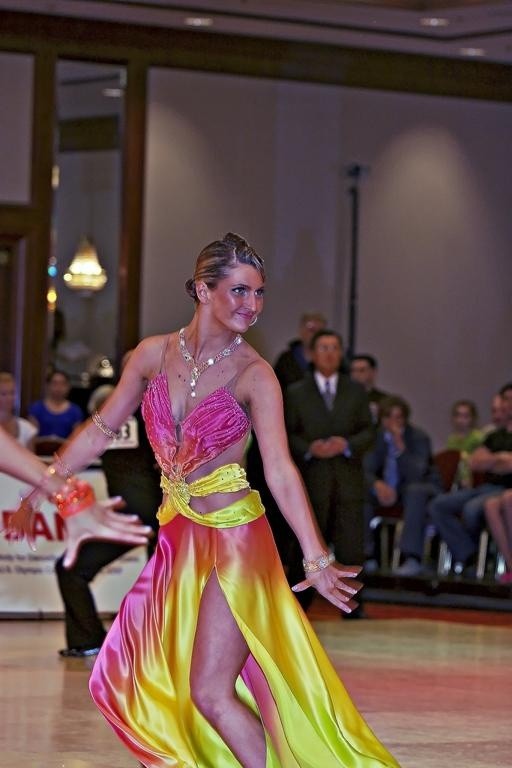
[372,449,506,582]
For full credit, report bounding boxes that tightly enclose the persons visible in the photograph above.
[275,314,511,619]
[1,428,151,567]
[0,231,400,768]
[1,352,161,658]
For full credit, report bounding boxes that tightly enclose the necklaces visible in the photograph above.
[179,328,243,397]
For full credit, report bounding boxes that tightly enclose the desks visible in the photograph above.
[0,470,148,621]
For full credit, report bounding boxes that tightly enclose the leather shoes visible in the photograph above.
[60,643,104,657]
[341,596,365,619]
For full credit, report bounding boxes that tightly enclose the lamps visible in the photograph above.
[63,235,108,291]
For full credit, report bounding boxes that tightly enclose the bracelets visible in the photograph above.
[52,477,96,520]
[51,451,73,482]
[303,549,336,572]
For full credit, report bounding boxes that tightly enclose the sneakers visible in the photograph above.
[392,556,420,577]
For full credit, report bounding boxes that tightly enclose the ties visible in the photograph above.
[322,380,332,409]
